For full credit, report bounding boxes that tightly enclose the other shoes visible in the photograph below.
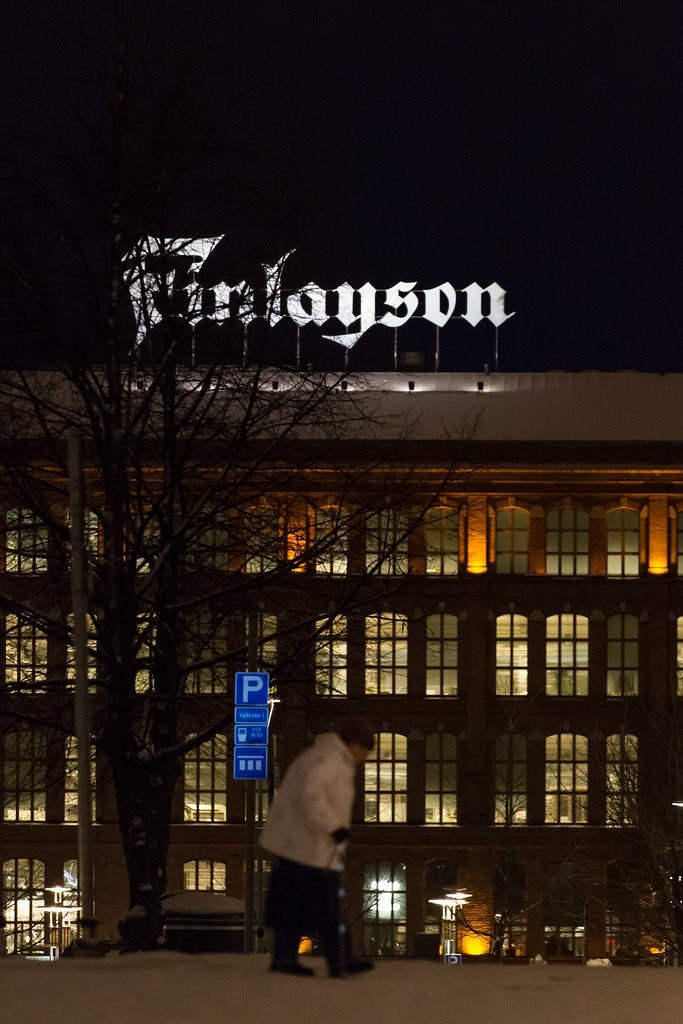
[328,958,375,977]
[270,961,314,977]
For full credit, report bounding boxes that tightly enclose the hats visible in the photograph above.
[335,719,374,747]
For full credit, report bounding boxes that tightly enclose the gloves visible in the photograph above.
[332,828,350,844]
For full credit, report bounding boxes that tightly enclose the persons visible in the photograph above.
[258,717,375,979]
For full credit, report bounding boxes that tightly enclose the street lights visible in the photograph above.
[252,693,281,957]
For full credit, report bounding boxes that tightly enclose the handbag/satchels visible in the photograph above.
[262,881,314,934]
